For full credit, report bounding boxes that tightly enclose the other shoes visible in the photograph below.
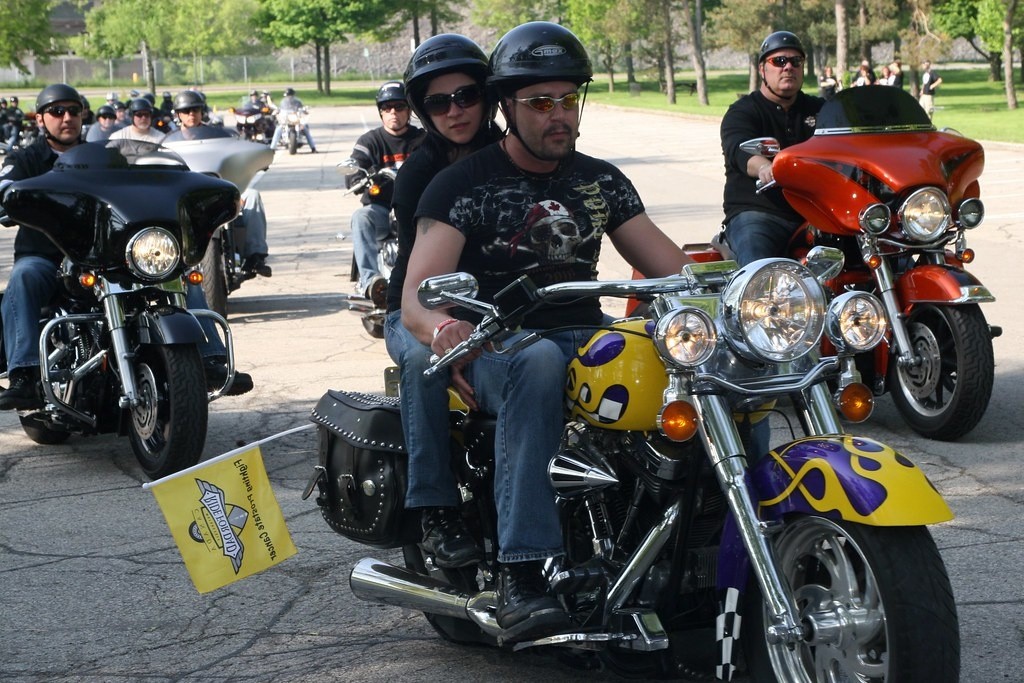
[367,276,388,310]
[312,147,318,153]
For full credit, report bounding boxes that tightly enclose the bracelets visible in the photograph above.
[433,319,458,339]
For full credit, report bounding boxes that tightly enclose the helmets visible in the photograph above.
[376,81,406,105]
[759,31,805,63]
[485,21,594,87]
[36,84,83,113]
[79,88,298,120]
[0,97,17,103]
[403,33,497,143]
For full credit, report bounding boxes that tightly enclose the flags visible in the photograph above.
[152,445,299,595]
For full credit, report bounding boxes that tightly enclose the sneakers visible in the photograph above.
[0,368,42,410]
[421,506,484,568]
[495,560,566,630]
[204,357,253,396]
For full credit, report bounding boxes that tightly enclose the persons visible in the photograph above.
[0,88,318,277]
[720,31,915,269]
[384,22,771,630]
[819,59,943,121]
[345,82,427,310]
[0,84,255,397]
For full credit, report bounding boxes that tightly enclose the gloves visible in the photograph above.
[352,179,366,195]
[3,185,39,220]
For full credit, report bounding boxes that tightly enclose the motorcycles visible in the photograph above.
[303,247,959,682]
[0,96,316,480]
[335,151,408,340]
[628,84,1004,443]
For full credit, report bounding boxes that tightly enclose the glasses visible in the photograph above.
[382,103,405,112]
[423,82,485,115]
[134,113,149,117]
[178,107,198,114]
[511,90,580,112]
[764,56,804,68]
[43,106,81,116]
[102,115,112,119]
